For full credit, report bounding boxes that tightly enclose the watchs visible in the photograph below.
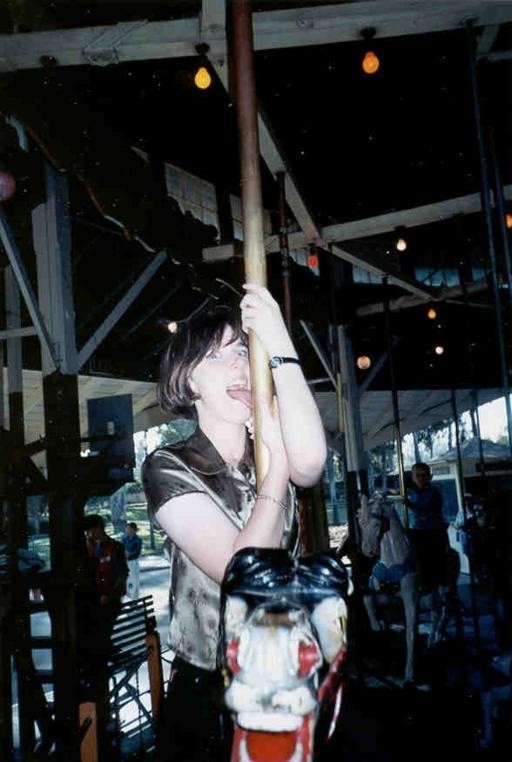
[265,350,310,369]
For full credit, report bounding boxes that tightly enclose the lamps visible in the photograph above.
[357,25,385,76]
[393,224,408,251]
[304,241,318,269]
[194,40,214,91]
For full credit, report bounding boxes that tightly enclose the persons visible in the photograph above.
[451,491,480,582]
[85,514,138,667]
[122,522,142,598]
[398,458,447,598]
[137,279,331,762]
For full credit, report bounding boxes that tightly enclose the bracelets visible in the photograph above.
[254,490,290,512]
